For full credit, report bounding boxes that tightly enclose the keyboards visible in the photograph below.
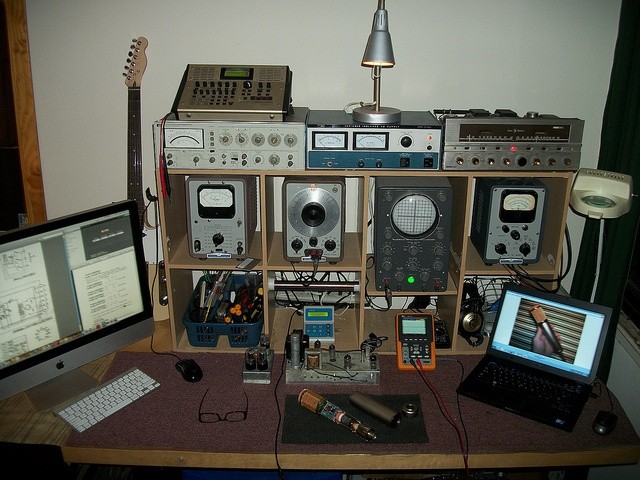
[54,367,161,435]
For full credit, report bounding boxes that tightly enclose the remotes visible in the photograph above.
[433,320,451,348]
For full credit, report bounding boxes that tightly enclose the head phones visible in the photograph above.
[460,282,485,347]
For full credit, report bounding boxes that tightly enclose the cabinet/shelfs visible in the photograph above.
[149,168,574,359]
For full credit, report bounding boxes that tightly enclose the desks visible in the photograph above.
[1,263,640,469]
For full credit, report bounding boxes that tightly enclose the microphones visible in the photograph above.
[526,303,566,360]
[296,388,381,442]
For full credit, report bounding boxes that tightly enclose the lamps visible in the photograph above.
[353,0,401,124]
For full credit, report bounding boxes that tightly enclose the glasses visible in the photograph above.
[199,387,247,423]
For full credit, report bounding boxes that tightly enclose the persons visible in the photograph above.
[532,324,561,357]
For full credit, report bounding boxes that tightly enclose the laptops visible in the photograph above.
[457,281,616,431]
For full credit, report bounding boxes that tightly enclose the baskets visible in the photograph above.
[182,273,263,348]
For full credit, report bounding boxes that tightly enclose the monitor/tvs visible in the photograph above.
[0,200,157,414]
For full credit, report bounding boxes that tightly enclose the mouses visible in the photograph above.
[175,357,204,382]
[591,410,618,436]
